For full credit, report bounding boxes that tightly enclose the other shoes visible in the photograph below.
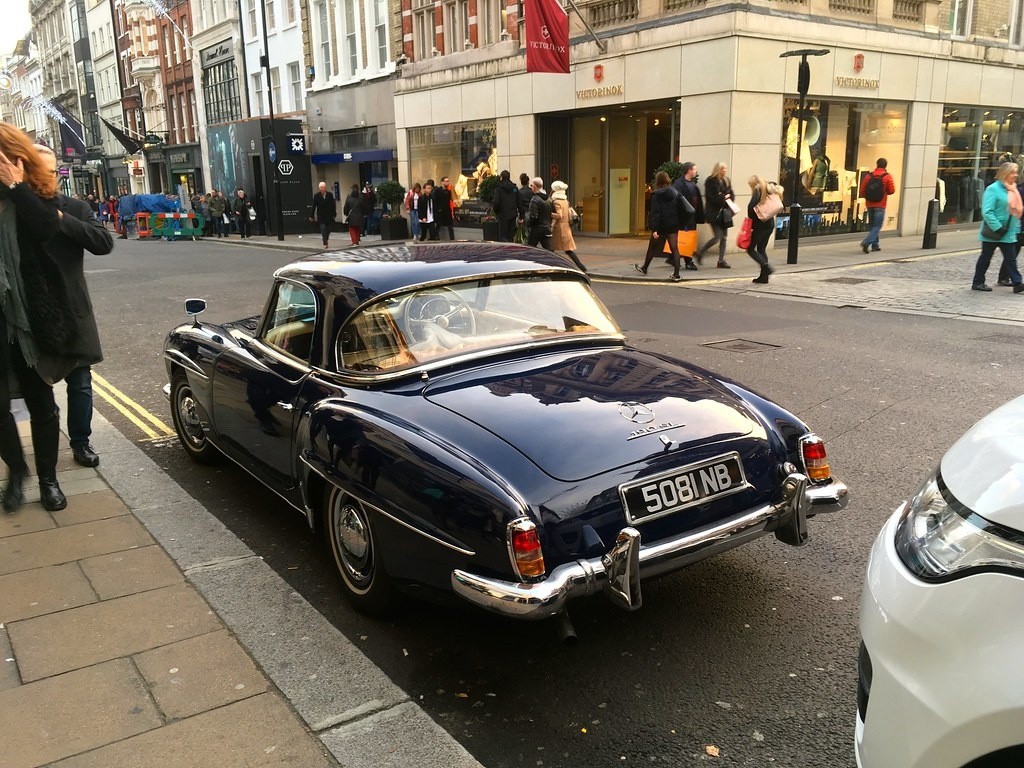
[685,261,698,270]
[972,283,992,291]
[348,242,359,247]
[1014,283,1024,293]
[753,265,774,283]
[861,242,869,254]
[1000,279,1012,286]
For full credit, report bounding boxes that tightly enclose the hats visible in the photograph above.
[551,180,569,191]
[362,187,371,193]
[532,177,543,187]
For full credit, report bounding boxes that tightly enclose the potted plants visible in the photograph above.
[376,181,408,241]
[479,175,500,241]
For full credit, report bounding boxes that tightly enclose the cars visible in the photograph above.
[162,238,852,644]
[852,390,1024,768]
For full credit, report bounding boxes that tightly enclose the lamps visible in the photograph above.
[430,47,438,54]
[501,29,508,37]
[465,39,472,46]
[994,24,1008,37]
[400,53,408,60]
[943,108,958,118]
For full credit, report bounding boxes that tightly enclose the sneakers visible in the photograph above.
[634,264,647,275]
[669,274,681,281]
[718,259,731,268]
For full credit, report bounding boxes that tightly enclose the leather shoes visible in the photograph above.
[73,445,99,467]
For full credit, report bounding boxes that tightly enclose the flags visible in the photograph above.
[56,102,86,155]
[100,117,146,155]
[525,0,571,73]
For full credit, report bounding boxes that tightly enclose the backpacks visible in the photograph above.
[866,172,887,201]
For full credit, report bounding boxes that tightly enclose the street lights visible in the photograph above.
[779,48,831,266]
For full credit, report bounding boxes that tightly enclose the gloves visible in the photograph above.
[999,226,1007,235]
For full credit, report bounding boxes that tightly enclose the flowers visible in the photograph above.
[480,215,498,224]
[381,209,404,222]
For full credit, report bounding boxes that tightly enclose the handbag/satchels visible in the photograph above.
[663,227,698,257]
[982,224,1003,240]
[722,208,733,229]
[681,195,695,224]
[248,207,256,220]
[754,194,784,223]
[737,217,753,249]
[568,206,580,226]
[223,213,230,224]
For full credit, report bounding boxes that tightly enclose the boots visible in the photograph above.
[30,419,67,510]
[0,412,29,512]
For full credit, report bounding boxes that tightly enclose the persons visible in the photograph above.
[665,163,705,270]
[231,188,252,240]
[859,158,895,254]
[343,182,375,247]
[492,170,588,272]
[405,177,455,241]
[309,182,337,248]
[808,154,831,195]
[71,193,119,230]
[695,163,735,268]
[0,121,68,512]
[746,175,783,284]
[635,173,682,280]
[191,189,231,237]
[972,162,1024,293]
[33,143,114,466]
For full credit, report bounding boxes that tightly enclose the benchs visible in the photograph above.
[270,293,454,360]
